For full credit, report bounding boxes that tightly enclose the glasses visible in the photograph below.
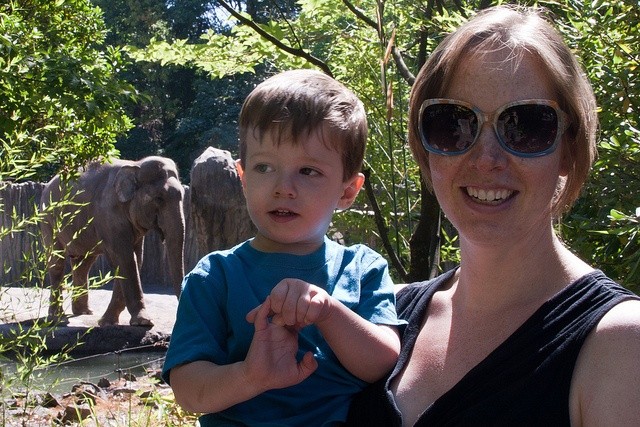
[417,98,573,158]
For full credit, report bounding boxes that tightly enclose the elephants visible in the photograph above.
[39,156,186,327]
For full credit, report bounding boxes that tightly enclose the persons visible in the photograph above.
[162,69,409,427]
[331,0,640,426]
